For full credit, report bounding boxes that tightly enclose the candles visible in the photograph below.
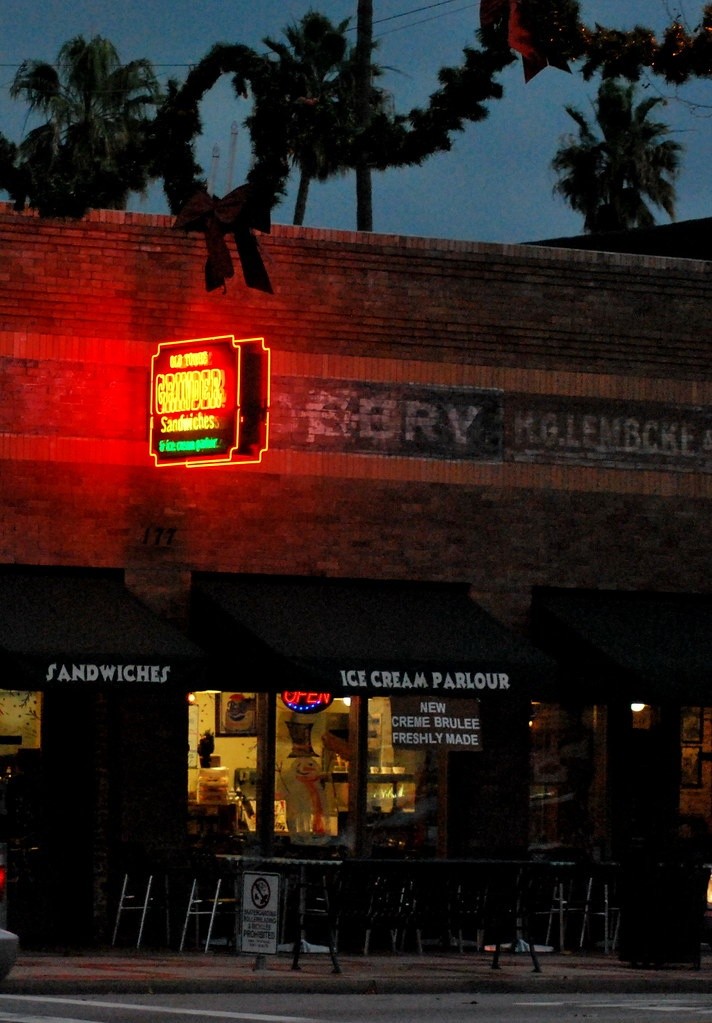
[369,765,379,775]
[380,766,393,775]
[394,766,404,775]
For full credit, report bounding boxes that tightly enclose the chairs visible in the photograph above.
[111,861,625,954]
